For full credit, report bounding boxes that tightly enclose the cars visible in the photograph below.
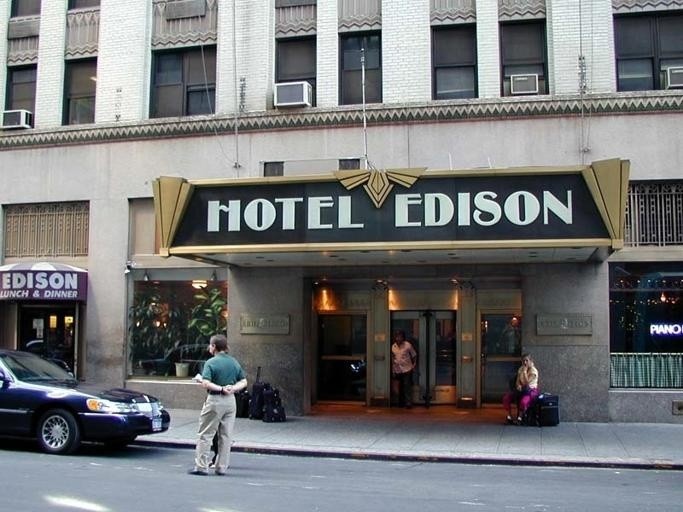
[138,342,213,377]
[1,348,171,454]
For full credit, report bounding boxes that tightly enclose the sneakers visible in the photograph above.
[516,420,524,425]
[188,462,227,475]
[504,420,513,424]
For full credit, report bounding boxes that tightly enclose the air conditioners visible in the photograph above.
[0,109,32,130]
[510,73,538,94]
[665,67,683,89]
[273,81,313,108]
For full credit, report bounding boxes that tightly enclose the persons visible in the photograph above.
[41,325,73,363]
[392,333,418,409]
[208,343,231,468]
[189,335,249,475]
[503,354,539,425]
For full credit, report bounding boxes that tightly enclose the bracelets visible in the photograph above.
[220,385,224,393]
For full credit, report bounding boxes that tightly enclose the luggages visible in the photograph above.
[536,392,559,427]
[235,367,286,422]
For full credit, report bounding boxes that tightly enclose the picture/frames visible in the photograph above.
[175,362,189,377]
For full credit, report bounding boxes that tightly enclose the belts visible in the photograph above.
[207,389,222,394]
